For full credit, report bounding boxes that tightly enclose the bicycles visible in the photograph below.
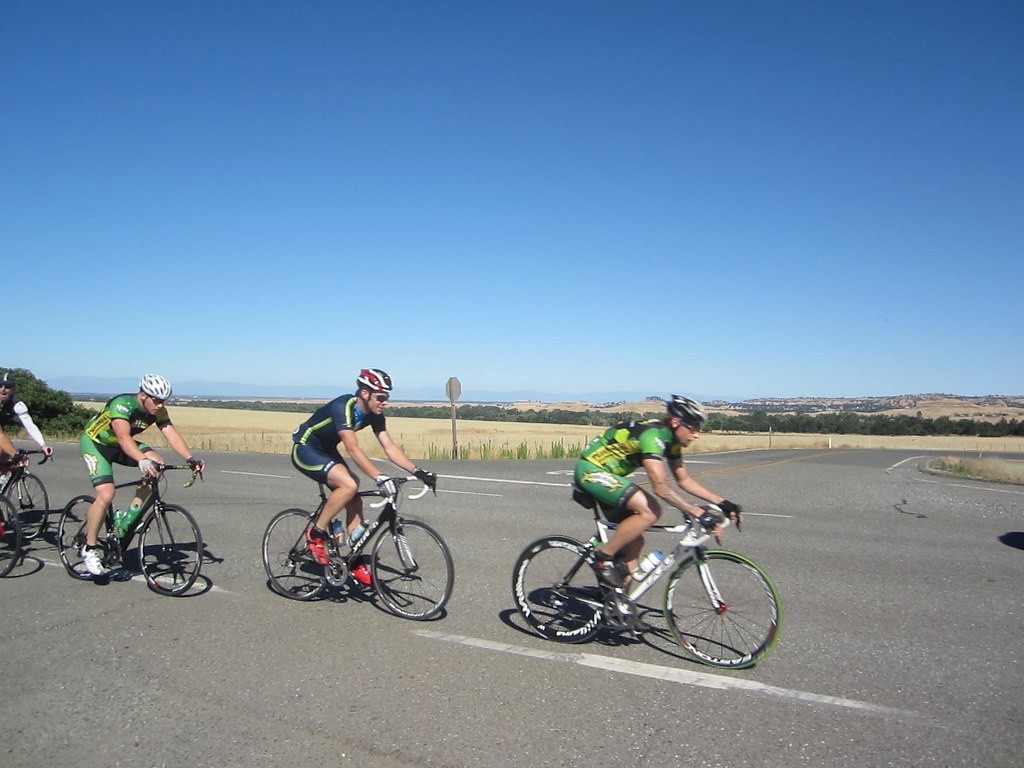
[510,484,782,668]
[259,471,458,623]
[59,456,207,599]
[0,445,53,575]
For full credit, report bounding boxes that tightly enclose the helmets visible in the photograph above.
[138,373,172,400]
[665,394,708,430]
[356,368,392,393]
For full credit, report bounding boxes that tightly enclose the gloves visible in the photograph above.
[376,474,397,498]
[413,467,438,496]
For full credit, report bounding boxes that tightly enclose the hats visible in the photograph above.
[0,372,15,387]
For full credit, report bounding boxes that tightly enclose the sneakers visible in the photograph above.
[592,550,624,589]
[634,619,652,632]
[134,520,151,534]
[80,544,103,575]
[305,528,328,565]
[352,564,373,586]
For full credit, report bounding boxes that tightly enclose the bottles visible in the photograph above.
[331,519,351,557]
[119,504,142,532]
[345,519,370,547]
[113,509,126,539]
[0,474,9,493]
[631,549,665,583]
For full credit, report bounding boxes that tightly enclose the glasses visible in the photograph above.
[371,394,390,402]
[149,397,163,404]
[680,423,701,434]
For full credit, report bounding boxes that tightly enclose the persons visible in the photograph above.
[573,395,744,632]
[291,368,437,585]
[0,371,53,536]
[80,374,205,576]
